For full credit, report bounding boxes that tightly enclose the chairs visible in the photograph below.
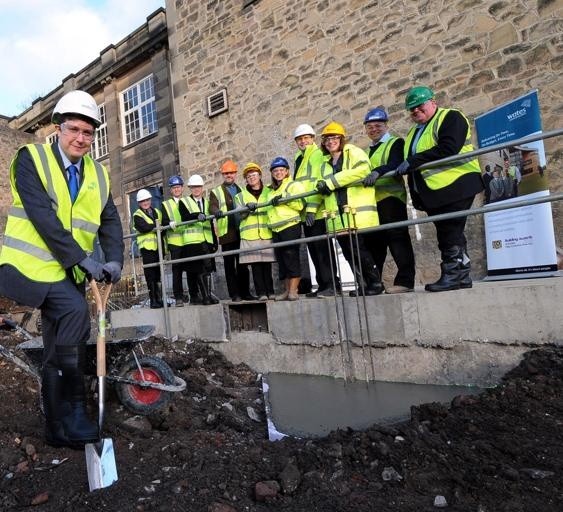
[306,286,414,296]
[176,298,184,307]
[231,294,276,300]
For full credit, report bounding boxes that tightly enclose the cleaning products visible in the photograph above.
[16,317,188,419]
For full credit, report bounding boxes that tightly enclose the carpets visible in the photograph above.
[196,201,202,212]
[68,165,78,204]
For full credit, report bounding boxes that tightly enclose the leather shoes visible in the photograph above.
[305,212,314,227]
[169,221,178,232]
[316,160,410,195]
[79,257,121,284]
[198,195,282,222]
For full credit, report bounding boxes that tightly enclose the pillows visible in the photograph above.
[221,158,288,177]
[406,86,433,109]
[364,109,387,124]
[295,122,346,140]
[51,90,102,128]
[136,174,203,202]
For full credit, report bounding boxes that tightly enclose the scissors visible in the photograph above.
[85,279,119,493]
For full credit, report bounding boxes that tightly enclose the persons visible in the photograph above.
[0,88,126,454]
[481,160,521,206]
[316,121,385,298]
[208,160,256,302]
[265,158,308,301]
[179,172,221,304]
[291,123,343,299]
[131,186,165,310]
[360,108,416,294]
[161,175,193,309]
[233,162,280,302]
[392,85,484,292]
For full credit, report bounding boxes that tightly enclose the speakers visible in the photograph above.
[275,277,301,300]
[147,280,171,308]
[41,367,99,445]
[425,245,472,291]
[198,274,218,304]
